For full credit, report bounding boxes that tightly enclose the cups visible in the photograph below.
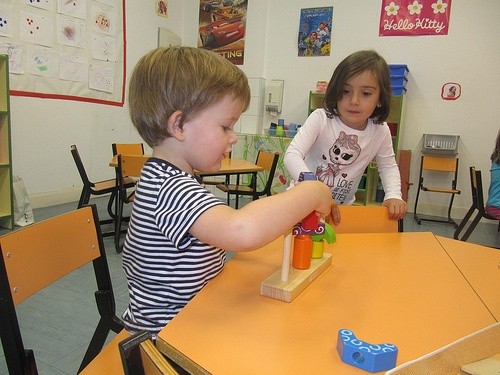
[269,118,302,138]
[311,240,324,259]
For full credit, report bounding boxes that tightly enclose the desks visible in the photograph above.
[155,231,500,375]
[194,158,263,200]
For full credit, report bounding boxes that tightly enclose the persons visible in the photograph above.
[122,46,333,340]
[486,130,500,219]
[284,50,407,221]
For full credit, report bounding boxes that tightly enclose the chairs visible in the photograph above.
[200,150,279,209]
[414,155,460,228]
[327,206,403,233]
[0,143,179,375]
[454,166,500,242]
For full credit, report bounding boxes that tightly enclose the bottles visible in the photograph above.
[292,234,311,270]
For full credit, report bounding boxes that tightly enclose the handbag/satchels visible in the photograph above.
[11,177,34,227]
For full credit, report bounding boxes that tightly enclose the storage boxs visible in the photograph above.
[388,64,408,94]
[421,134,461,157]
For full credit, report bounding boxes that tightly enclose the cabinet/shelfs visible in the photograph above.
[0,54,14,229]
[307,89,403,207]
